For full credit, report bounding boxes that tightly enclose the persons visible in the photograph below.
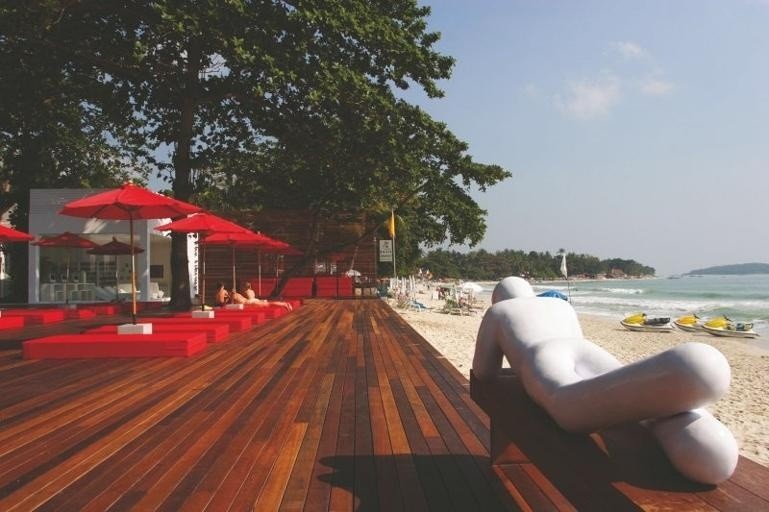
[393,285,465,307]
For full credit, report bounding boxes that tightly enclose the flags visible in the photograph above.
[388,213,396,238]
[560,256,568,280]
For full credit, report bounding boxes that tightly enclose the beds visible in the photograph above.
[82,323,231,343]
[0,309,64,330]
[28,303,122,320]
[122,302,164,314]
[139,300,301,332]
[23,333,207,357]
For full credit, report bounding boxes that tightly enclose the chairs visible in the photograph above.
[394,292,435,313]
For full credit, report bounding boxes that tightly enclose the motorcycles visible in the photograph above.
[701,315,762,341]
[618,311,675,333]
[672,313,708,332]
